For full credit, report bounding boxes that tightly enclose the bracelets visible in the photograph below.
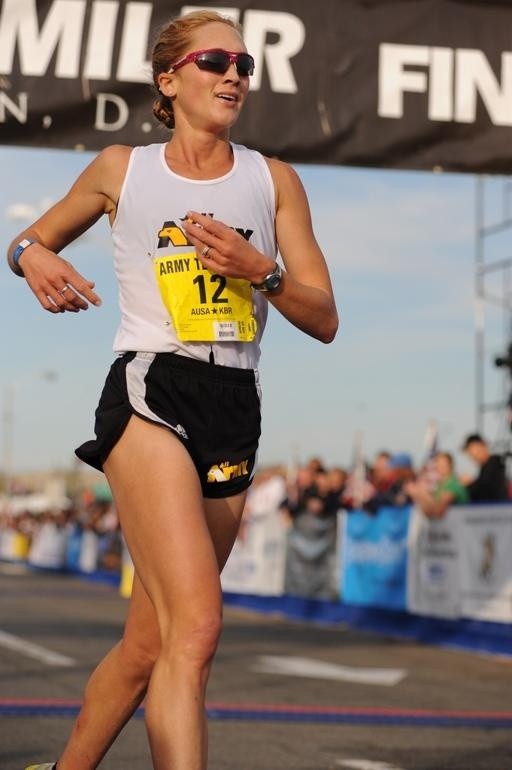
[13,238,35,267]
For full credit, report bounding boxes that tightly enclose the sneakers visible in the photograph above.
[26,762,57,769]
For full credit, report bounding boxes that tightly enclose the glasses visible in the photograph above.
[167,49,255,77]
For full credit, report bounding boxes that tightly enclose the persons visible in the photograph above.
[6,435,506,534]
[7,11,338,769]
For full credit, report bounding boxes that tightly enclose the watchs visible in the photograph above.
[249,262,282,293]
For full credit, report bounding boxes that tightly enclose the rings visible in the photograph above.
[61,286,68,293]
[202,247,209,255]
